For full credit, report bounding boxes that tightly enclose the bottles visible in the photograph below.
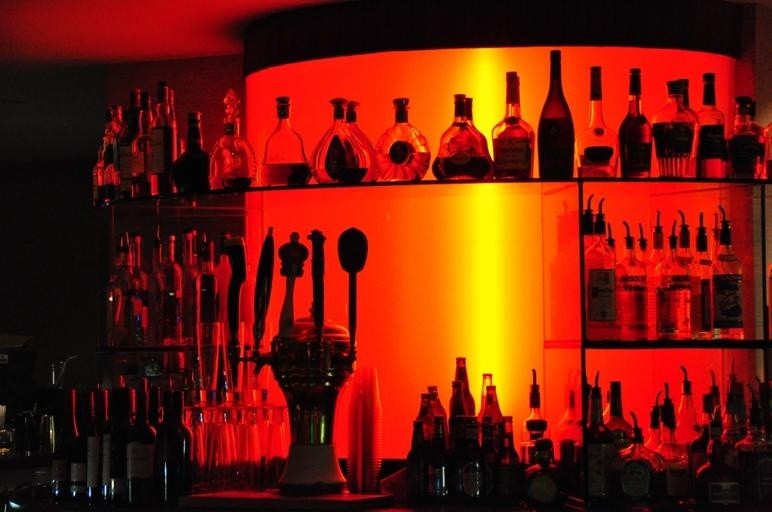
[575,65,620,178]
[94,83,210,200]
[727,95,772,180]
[431,94,494,180]
[650,78,699,179]
[538,48,575,179]
[374,97,431,181]
[491,70,536,179]
[618,66,653,177]
[699,71,726,178]
[257,93,312,186]
[210,88,257,190]
[404,357,771,511]
[311,96,378,186]
[350,362,387,488]
[582,193,744,339]
[35,229,222,500]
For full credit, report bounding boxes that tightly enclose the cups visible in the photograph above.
[206,321,291,491]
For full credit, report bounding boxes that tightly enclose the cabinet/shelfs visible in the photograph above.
[64,173,772,477]
